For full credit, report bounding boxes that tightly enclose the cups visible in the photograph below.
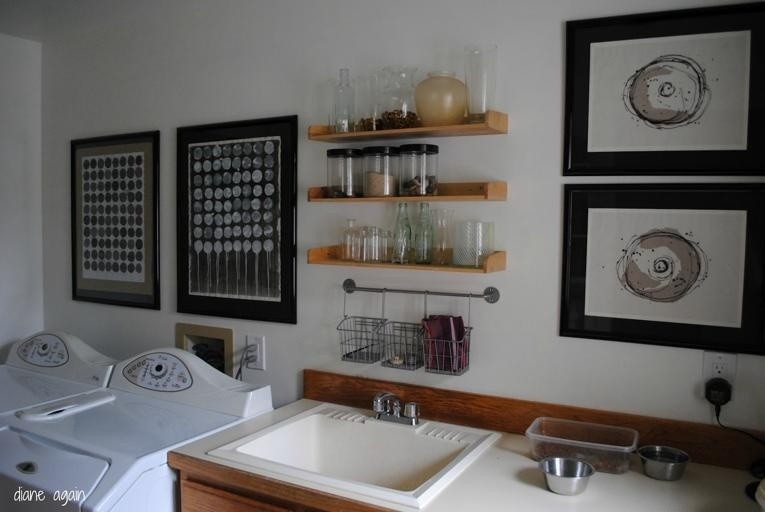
[342,216,391,262]
[450,217,497,268]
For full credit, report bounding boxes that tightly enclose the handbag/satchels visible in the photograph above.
[422,314,469,371]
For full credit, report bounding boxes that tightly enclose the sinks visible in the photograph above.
[206,405,503,512]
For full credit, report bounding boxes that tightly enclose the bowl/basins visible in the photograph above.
[637,443,691,481]
[538,454,596,495]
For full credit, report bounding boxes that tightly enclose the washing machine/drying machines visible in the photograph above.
[1,331,116,413]
[0,348,275,512]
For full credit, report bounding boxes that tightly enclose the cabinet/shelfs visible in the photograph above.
[306,107,510,276]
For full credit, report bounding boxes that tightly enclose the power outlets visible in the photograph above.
[245,335,265,370]
[700,351,738,402]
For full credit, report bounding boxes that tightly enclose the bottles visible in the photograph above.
[414,201,435,263]
[397,143,439,196]
[415,70,469,125]
[461,36,499,123]
[362,144,398,198]
[430,204,456,264]
[353,76,376,130]
[325,147,364,199]
[391,201,413,263]
[331,70,357,133]
[378,65,419,127]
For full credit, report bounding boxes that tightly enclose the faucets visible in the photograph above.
[373,390,402,419]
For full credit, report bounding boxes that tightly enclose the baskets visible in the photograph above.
[376,321,425,371]
[336,316,389,364]
[423,327,471,376]
